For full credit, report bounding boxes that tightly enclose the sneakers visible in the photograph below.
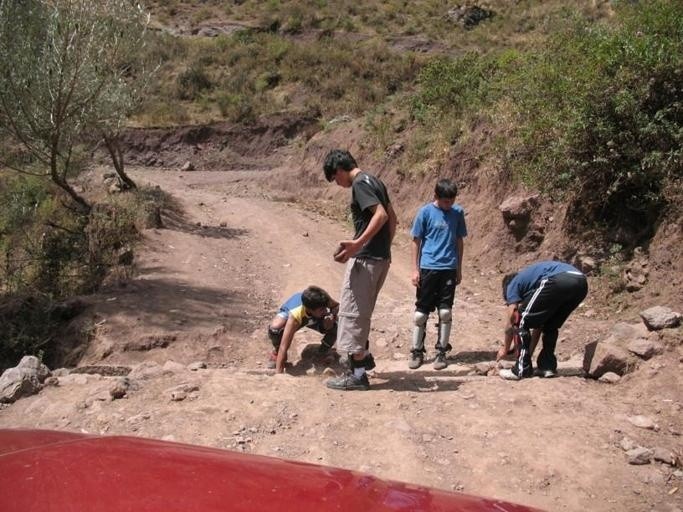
[499,367,519,381]
[325,369,370,392]
[432,354,448,371]
[533,369,555,378]
[339,354,377,372]
[267,349,277,368]
[407,352,424,370]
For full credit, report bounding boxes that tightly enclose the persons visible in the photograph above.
[408,179,468,369]
[267,286,340,373]
[322,148,397,390]
[497,262,589,381]
